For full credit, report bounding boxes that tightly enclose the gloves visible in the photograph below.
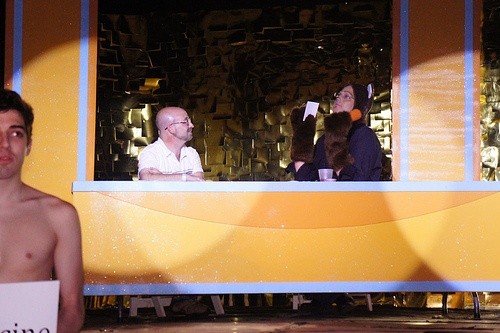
[289,106,317,163]
[323,111,355,170]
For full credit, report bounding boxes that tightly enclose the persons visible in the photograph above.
[286,82,383,312]
[137,107,211,315]
[0,88,85,332]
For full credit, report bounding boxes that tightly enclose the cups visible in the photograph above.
[319,169,333,182]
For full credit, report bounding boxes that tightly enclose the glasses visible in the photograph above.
[335,93,355,102]
[164,118,191,130]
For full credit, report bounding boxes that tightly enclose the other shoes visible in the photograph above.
[171,300,209,313]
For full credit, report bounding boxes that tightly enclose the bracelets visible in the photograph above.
[182,173,187,182]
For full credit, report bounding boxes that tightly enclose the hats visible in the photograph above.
[349,83,374,120]
